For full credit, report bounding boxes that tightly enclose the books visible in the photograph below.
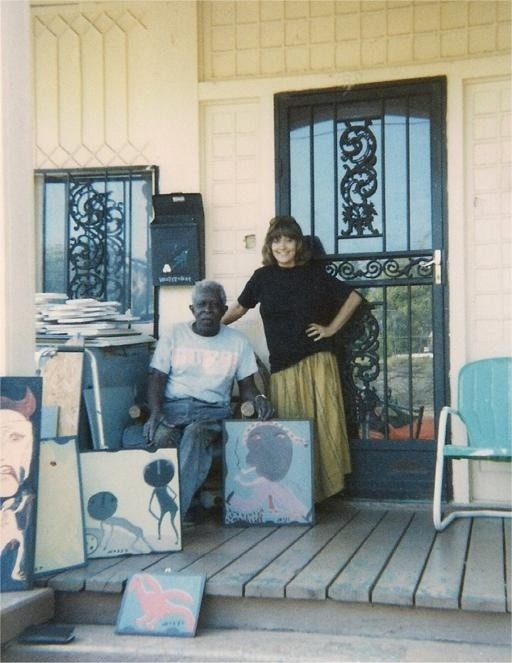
[19,623,75,643]
[34,293,143,339]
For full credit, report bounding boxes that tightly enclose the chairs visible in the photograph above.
[432,357,512,531]
[134,355,270,522]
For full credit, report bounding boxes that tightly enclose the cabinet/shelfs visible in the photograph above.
[35,342,148,454]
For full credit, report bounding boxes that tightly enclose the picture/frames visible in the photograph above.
[0,377,41,592]
[220,417,316,527]
[114,570,207,637]
[32,436,88,578]
[79,448,183,559]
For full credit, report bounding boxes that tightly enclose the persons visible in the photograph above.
[220,215,362,506]
[123,281,275,525]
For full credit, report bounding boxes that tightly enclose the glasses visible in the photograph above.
[269,216,295,226]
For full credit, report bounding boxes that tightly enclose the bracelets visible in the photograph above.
[255,393,267,401]
